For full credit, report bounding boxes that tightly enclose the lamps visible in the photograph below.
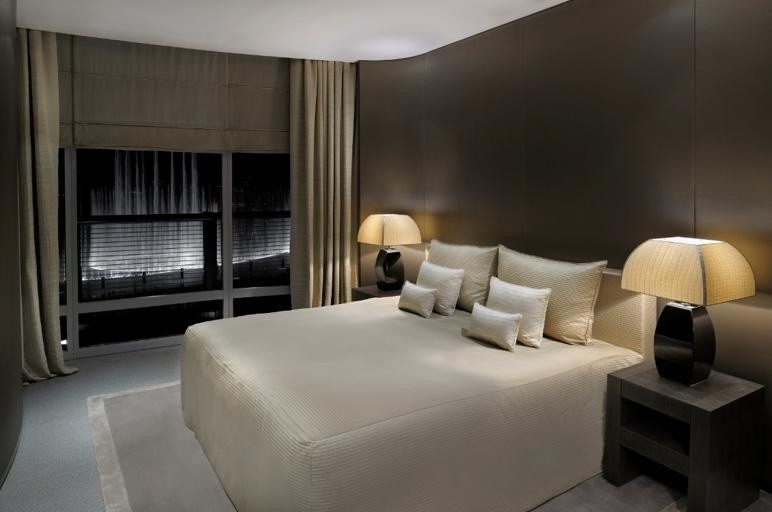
[358,209,422,293]
[620,233,758,385]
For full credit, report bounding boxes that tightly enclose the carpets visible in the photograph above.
[83,371,685,510]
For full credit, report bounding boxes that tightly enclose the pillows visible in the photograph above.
[416,262,465,316]
[400,280,436,320]
[486,271,552,351]
[429,238,494,310]
[463,302,523,352]
[492,242,609,346]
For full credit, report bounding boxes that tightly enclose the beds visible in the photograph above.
[181,243,659,510]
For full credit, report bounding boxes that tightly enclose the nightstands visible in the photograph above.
[605,352,770,511]
[352,279,403,304]
